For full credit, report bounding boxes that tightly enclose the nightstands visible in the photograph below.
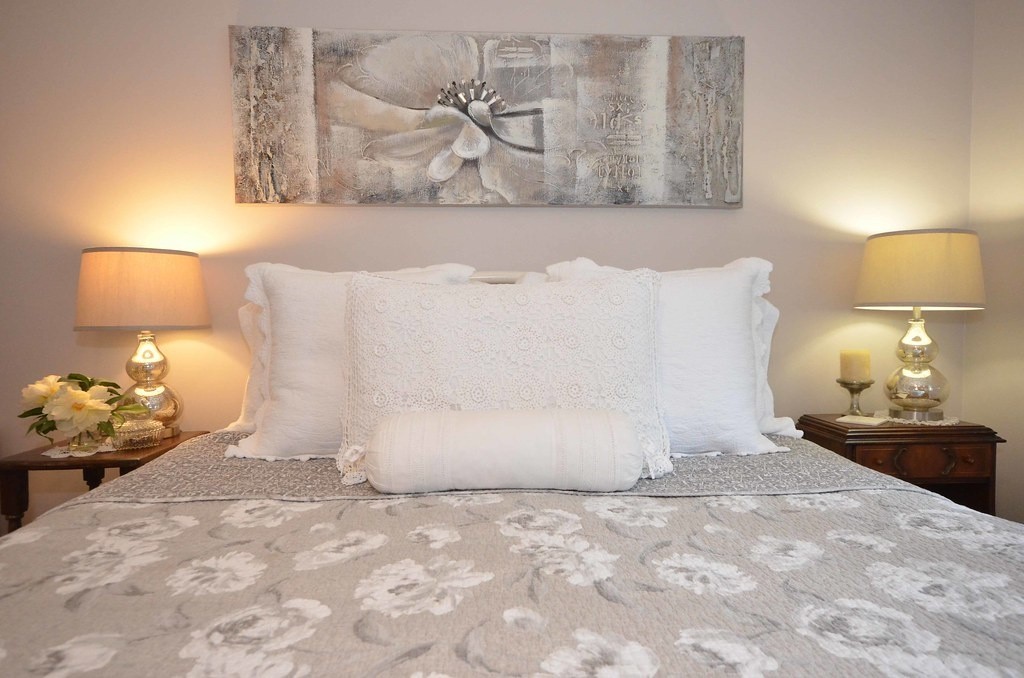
[793,412,1007,514]
[0,430,210,534]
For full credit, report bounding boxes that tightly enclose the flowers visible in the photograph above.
[17,373,152,448]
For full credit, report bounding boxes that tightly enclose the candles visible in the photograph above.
[839,348,873,383]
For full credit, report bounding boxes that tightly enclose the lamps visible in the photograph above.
[74,246,213,440]
[853,228,989,422]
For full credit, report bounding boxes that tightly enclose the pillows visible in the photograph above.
[225,262,475,461]
[230,302,266,432]
[344,410,644,491]
[546,257,789,457]
[337,271,660,493]
[758,295,804,438]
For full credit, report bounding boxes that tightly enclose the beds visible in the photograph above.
[0,269,1024,678]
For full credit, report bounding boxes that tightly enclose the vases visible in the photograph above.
[69,430,99,454]
